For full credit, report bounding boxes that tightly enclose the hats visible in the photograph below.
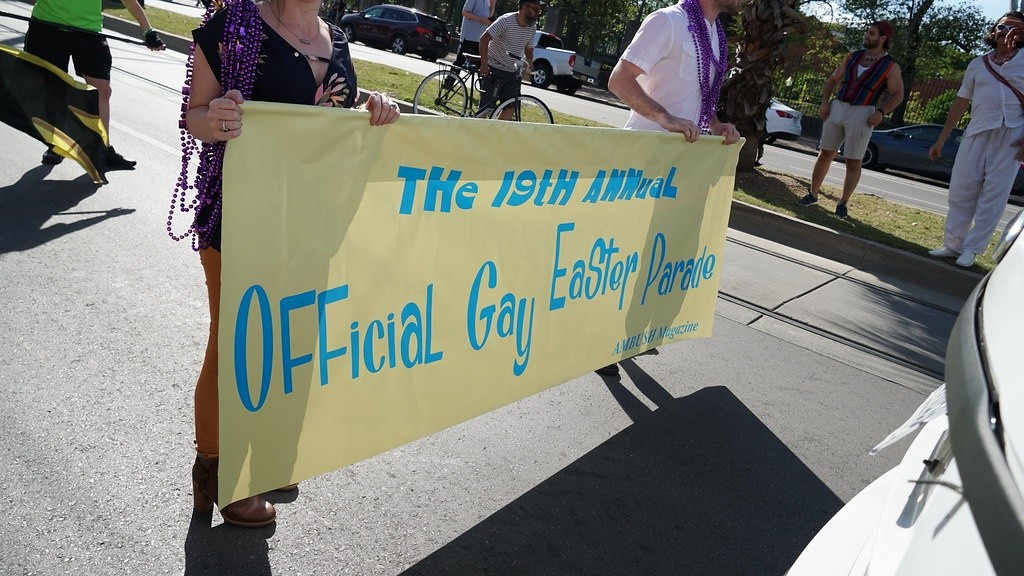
[519,0,546,9]
[872,20,893,52]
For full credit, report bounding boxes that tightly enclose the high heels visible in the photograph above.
[192,450,276,526]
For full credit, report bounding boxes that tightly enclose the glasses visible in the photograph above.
[996,24,1015,32]
[524,4,543,15]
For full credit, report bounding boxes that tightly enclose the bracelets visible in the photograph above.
[877,109,885,117]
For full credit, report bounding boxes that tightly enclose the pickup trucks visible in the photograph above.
[530,30,603,95]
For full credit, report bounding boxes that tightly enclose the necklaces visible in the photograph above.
[167,0,266,253]
[682,0,729,135]
[268,3,319,45]
[862,49,884,63]
[997,48,1009,57]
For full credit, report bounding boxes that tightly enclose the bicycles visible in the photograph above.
[413,51,554,125]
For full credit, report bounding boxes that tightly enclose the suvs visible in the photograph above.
[339,4,451,62]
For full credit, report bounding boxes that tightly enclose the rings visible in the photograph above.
[222,120,228,132]
[390,103,397,109]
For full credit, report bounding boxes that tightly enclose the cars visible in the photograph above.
[763,97,803,144]
[783,209,1024,576]
[841,123,1024,198]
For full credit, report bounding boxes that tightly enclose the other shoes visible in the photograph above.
[42,147,65,164]
[956,251,977,267]
[928,246,960,259]
[596,365,618,374]
[442,83,454,93]
[106,145,136,169]
[834,202,848,220]
[797,191,818,206]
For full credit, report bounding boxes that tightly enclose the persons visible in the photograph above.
[479,0,542,121]
[27,0,168,173]
[597,0,747,376]
[186,0,401,525]
[928,11,1024,268]
[797,22,906,220]
[441,0,495,90]
